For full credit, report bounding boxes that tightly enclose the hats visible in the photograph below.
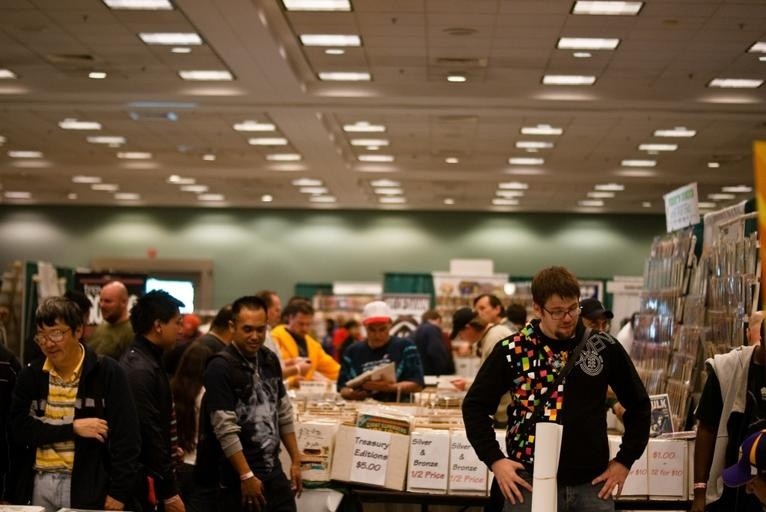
[581,296,614,321]
[361,301,392,325]
[720,427,766,487]
[449,308,478,341]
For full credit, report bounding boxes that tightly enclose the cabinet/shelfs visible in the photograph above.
[0,256,74,371]
[282,199,766,512]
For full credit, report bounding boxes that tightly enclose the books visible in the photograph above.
[628,264,765,442]
[279,359,466,482]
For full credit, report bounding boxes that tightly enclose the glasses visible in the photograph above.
[539,299,583,321]
[31,326,76,345]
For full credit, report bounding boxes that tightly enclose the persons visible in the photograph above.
[2,282,303,512]
[691,311,765,511]
[256,289,528,404]
[461,266,652,511]
[580,299,641,424]
